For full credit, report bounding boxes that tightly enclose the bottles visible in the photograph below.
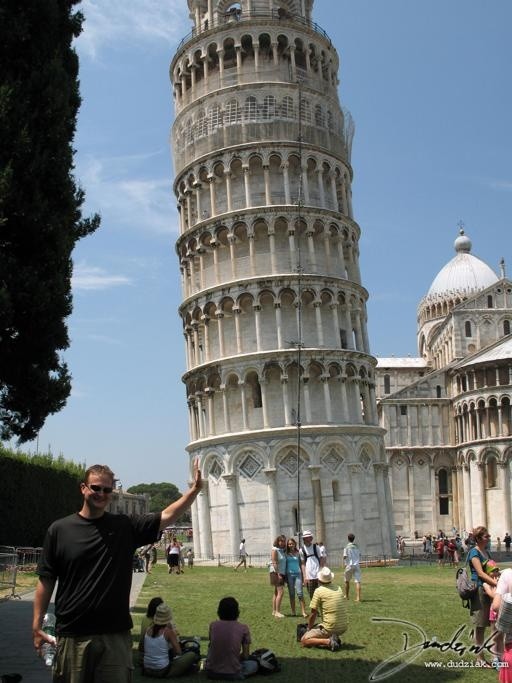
[40,614,57,667]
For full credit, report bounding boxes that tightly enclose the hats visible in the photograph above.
[481,558,499,575]
[153,602,173,626]
[302,530,314,538]
[317,566,334,583]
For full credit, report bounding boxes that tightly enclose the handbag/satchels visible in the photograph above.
[297,623,317,642]
[494,599,512,634]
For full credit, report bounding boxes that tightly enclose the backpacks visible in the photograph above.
[249,648,282,676]
[169,640,201,676]
[456,548,485,609]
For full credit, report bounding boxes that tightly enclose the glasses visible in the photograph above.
[89,485,114,494]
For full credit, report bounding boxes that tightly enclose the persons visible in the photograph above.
[31,457,204,682]
[132,525,512,683]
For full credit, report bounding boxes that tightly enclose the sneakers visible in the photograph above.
[272,612,285,618]
[329,634,340,652]
